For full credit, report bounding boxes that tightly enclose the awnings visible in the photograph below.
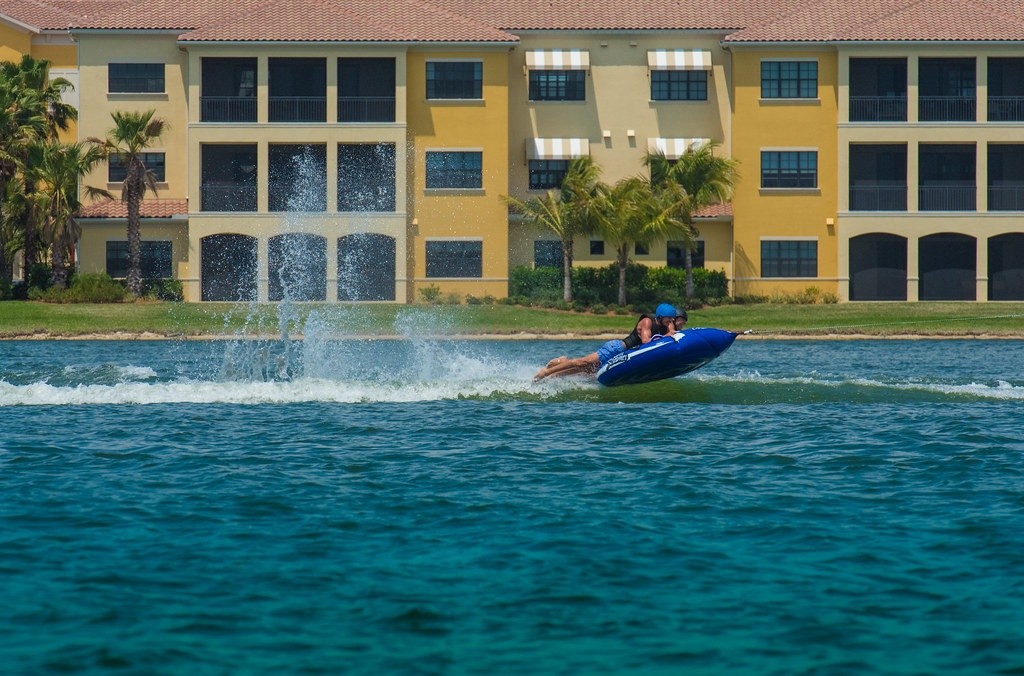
[526,136,588,160]
[647,137,712,160]
[647,48,713,71]
[523,49,591,72]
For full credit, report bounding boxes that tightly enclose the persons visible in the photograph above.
[532,302,688,382]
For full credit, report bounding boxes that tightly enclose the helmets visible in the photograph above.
[676,307,688,321]
[656,304,676,318]
